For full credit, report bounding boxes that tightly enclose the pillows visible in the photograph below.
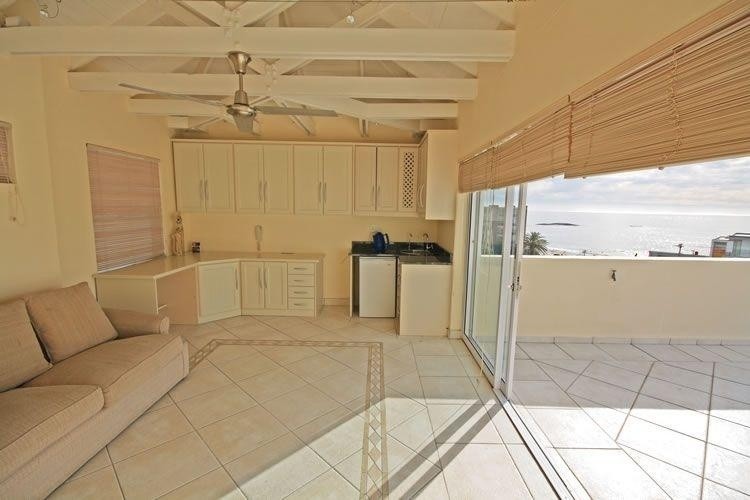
[2,298,53,393]
[27,281,118,363]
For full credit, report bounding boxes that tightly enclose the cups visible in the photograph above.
[191,242,201,255]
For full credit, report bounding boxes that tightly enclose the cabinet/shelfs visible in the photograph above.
[418,130,460,221]
[353,142,418,218]
[294,141,355,215]
[172,138,236,215]
[350,253,395,318]
[93,253,324,325]
[396,259,450,336]
[234,139,294,213]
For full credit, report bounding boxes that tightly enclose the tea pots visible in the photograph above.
[373,232,389,253]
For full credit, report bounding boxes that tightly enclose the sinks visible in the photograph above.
[400,249,431,256]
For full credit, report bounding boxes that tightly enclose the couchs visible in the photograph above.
[1,282,190,500]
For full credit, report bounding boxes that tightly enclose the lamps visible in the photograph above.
[37,1,60,19]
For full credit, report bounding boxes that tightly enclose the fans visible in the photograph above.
[117,49,336,133]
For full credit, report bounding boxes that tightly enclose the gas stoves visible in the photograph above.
[350,243,398,258]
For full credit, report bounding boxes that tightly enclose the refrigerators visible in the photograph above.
[358,257,396,317]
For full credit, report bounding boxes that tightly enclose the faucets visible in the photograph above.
[407,232,413,248]
[423,233,435,250]
